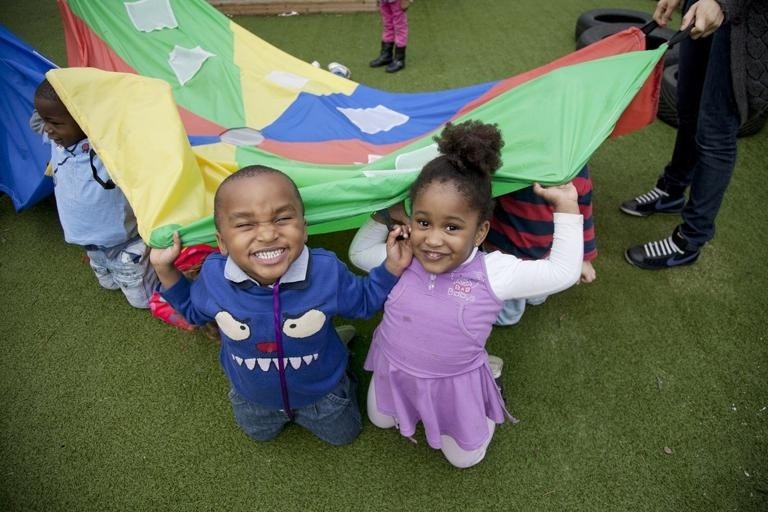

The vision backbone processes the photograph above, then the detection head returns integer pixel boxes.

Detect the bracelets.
[374,209,391,222]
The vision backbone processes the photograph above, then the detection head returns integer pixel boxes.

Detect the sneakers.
[619,187,686,218]
[336,325,357,347]
[624,224,706,271]
[486,355,505,380]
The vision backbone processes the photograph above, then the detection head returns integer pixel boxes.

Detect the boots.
[385,45,406,73]
[369,41,392,67]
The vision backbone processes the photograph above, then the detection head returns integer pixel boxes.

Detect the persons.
[348,120,585,467]
[480,161,598,327]
[28,78,161,310]
[149,164,413,445]
[618,1,767,270]
[368,1,414,73]
[311,59,353,80]
